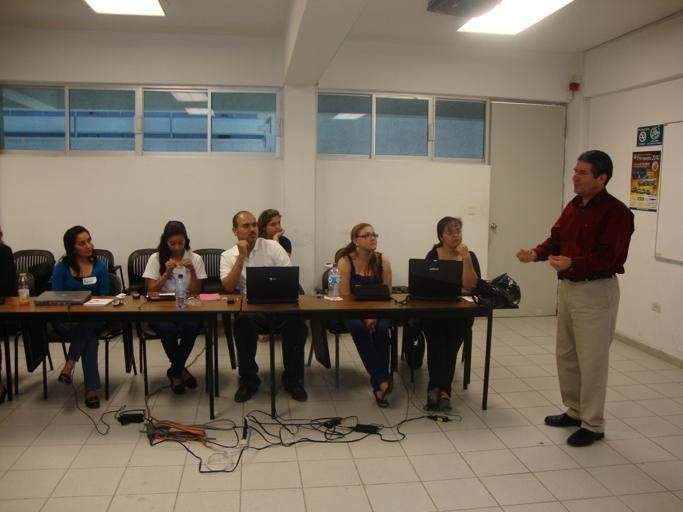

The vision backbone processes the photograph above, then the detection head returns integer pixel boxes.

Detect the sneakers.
[166,368,186,394]
[179,365,198,390]
[234,379,260,403]
[282,378,308,402]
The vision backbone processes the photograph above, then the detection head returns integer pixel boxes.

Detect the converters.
[354,423,379,434]
[432,413,448,423]
[323,417,341,428]
[117,412,144,424]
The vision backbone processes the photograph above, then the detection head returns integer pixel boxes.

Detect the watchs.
[567,261,576,272]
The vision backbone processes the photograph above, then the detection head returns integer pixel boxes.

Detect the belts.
[562,272,613,283]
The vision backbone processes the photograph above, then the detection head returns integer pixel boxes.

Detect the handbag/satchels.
[470,272,522,308]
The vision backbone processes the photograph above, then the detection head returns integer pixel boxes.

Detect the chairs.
[306,267,351,389]
[9,250,57,396]
[128,248,212,398]
[41,249,117,401]
[193,249,231,292]
[400,325,473,389]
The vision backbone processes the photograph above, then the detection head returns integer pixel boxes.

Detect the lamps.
[83,0,167,18]
[456,0,573,37]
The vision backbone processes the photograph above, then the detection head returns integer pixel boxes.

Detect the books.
[83,298,113,307]
[198,293,221,302]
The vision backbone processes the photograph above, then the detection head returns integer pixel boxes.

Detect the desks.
[242,294,520,420]
[0,294,242,420]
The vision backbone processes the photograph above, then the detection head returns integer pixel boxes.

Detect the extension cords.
[238,420,252,446]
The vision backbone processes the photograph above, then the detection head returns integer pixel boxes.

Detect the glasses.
[358,233,379,238]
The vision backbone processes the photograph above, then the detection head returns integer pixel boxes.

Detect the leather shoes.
[85,395,100,408]
[372,389,389,408]
[59,369,72,384]
[545,413,581,427]
[385,375,394,396]
[566,428,605,447]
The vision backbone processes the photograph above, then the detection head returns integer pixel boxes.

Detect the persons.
[219,210,309,404]
[425,216,482,411]
[516,151,635,448]
[142,220,208,396]
[257,208,292,258]
[0,228,18,407]
[336,223,395,408]
[52,226,109,409]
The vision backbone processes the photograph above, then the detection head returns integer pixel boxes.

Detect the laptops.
[245,266,300,304]
[34,290,92,306]
[408,258,462,302]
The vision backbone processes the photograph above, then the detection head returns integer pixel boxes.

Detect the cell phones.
[226,296,234,303]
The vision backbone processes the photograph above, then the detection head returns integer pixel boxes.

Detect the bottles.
[328,264,341,299]
[175,274,186,310]
[18,274,30,306]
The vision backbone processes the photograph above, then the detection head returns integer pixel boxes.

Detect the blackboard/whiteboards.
[654,120,683,264]
